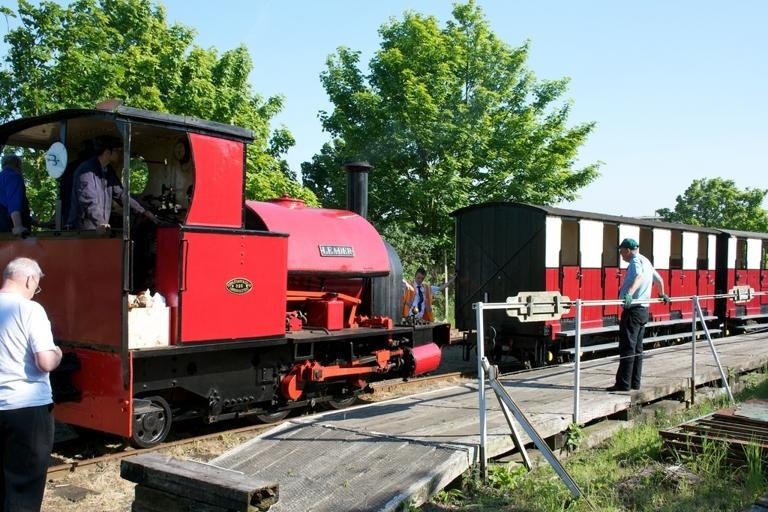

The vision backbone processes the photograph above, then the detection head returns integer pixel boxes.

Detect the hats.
[616,238,639,250]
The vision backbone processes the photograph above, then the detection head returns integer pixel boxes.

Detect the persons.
[0,154,35,238]
[0,257,64,512]
[68,138,158,231]
[409,266,456,319]
[606,238,673,391]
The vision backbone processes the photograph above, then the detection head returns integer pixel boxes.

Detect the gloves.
[659,294,671,305]
[624,294,633,308]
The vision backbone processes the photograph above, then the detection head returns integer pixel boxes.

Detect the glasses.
[32,277,42,295]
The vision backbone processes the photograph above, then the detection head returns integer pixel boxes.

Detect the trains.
[0,97,763,453]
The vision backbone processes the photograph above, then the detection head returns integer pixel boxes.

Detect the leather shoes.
[631,385,640,390]
[606,384,630,391]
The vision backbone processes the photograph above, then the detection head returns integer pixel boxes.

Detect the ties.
[417,285,423,312]
